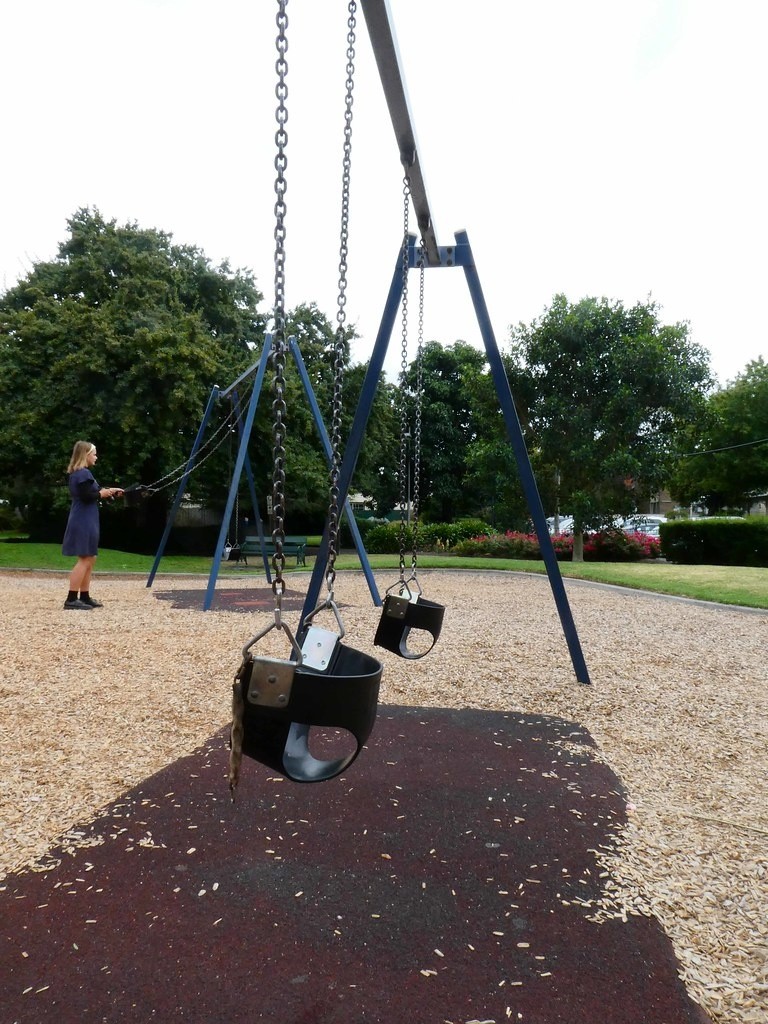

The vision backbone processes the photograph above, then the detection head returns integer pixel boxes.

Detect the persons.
[60,440,124,611]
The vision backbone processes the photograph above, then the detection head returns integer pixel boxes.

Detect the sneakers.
[83,598,103,608]
[63,599,93,610]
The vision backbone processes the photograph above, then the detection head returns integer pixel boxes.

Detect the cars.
[544,511,746,538]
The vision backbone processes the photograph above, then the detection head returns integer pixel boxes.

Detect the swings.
[373,163,445,659]
[117,370,264,503]
[224,0,382,793]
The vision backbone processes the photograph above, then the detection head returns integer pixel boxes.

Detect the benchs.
[240,535,309,568]
